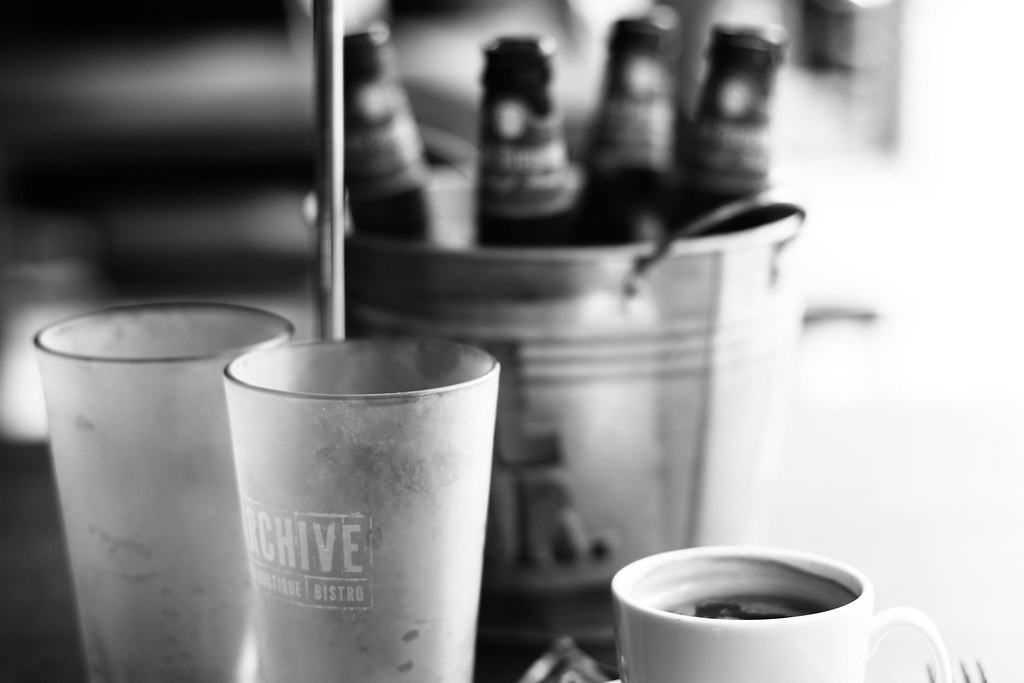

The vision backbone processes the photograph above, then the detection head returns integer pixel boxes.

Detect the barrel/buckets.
[301,174,803,650]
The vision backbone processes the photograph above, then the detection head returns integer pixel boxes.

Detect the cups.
[34,301,293,683]
[222,336,502,683]
[612,544,949,683]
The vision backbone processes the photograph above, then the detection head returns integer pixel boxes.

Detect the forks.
[924,658,991,682]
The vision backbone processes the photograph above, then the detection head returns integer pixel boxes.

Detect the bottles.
[473,35,587,244]
[679,22,788,236]
[342,26,458,239]
[585,16,676,244]
